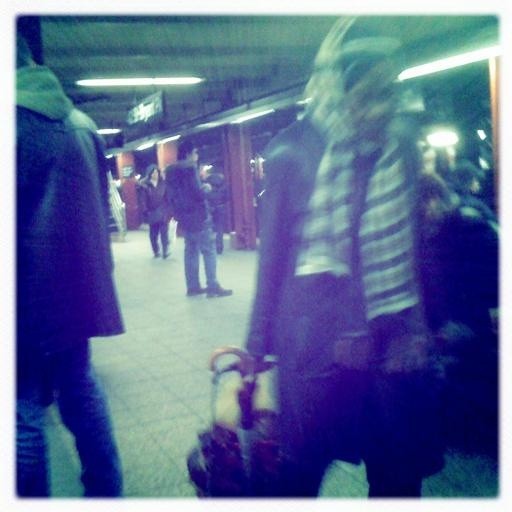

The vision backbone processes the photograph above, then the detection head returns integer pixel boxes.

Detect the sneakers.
[157,251,171,257]
[209,286,231,296]
[186,287,206,295]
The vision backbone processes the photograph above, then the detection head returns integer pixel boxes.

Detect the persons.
[138,165,171,258]
[420,163,500,470]
[245,15,427,499]
[166,141,232,297]
[14,14,127,497]
